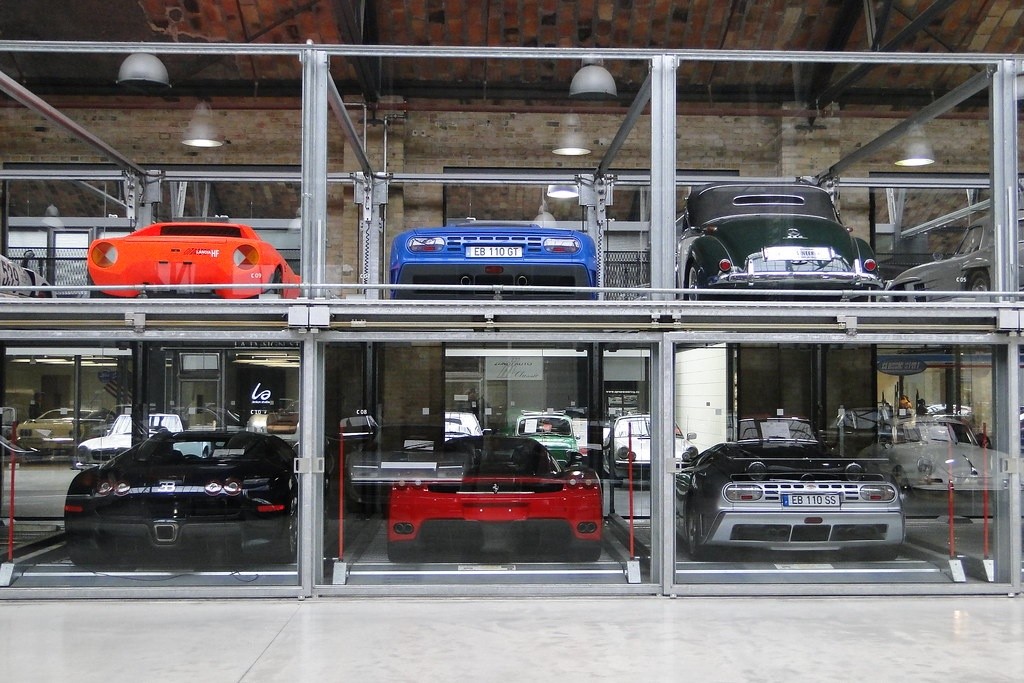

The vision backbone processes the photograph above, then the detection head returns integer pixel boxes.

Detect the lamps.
[115,52,171,95]
[550,114,591,156]
[894,125,935,165]
[181,101,224,148]
[568,59,616,101]
[534,201,554,221]
[545,184,580,198]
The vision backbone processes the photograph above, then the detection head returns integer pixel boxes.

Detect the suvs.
[734,413,832,458]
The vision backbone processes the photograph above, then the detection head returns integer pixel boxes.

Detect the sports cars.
[677,438,907,559]
[385,206,599,301]
[82,216,305,302]
[390,431,605,560]
[61,429,299,568]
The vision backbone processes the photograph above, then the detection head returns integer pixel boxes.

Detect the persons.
[974,433,991,449]
[899,395,912,409]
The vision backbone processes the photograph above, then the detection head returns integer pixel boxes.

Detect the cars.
[608,415,701,488]
[14,403,112,445]
[846,408,1008,517]
[886,194,1024,292]
[514,407,580,473]
[345,421,472,502]
[677,178,885,302]
[262,398,317,433]
[242,412,305,442]
[172,407,245,428]
[73,412,188,468]
[0,247,55,299]
